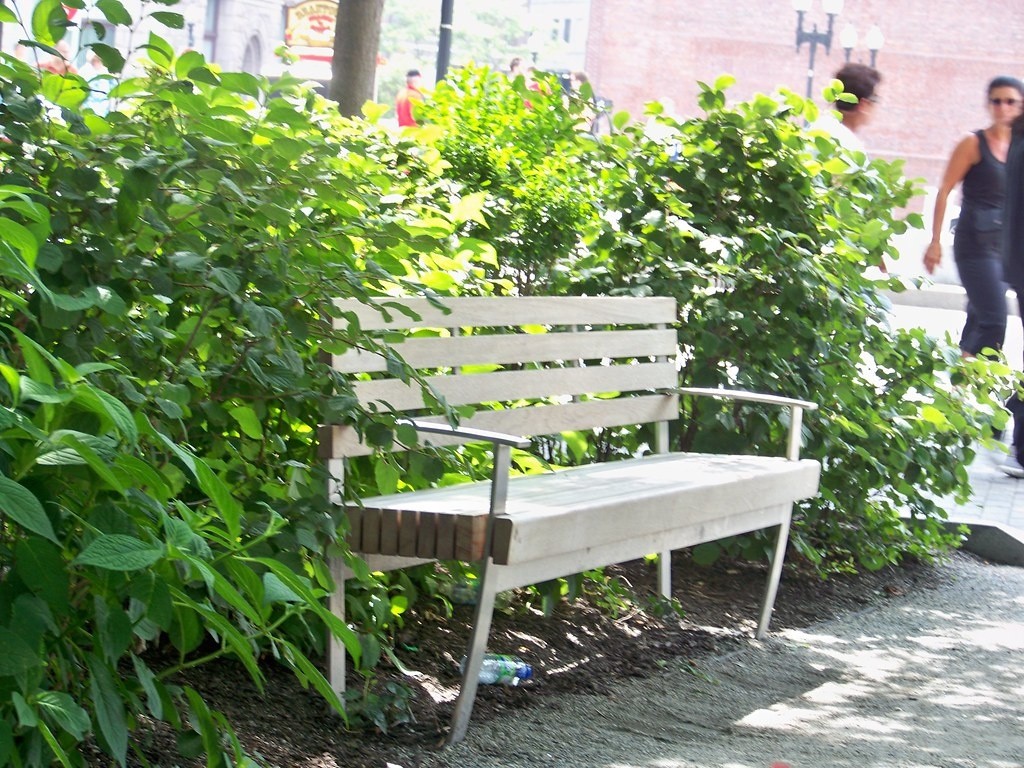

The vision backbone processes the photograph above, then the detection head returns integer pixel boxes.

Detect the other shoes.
[999,445,1024,478]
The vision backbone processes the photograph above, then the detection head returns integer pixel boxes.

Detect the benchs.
[324,294,821,754]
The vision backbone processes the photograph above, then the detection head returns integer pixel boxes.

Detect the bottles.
[459,653,536,686]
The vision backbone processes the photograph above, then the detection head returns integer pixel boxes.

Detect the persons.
[507,57,527,77]
[571,74,588,91]
[396,68,427,126]
[922,75,1024,475]
[822,63,883,164]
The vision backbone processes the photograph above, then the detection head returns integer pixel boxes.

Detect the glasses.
[863,94,881,105]
[988,97,1022,106]
